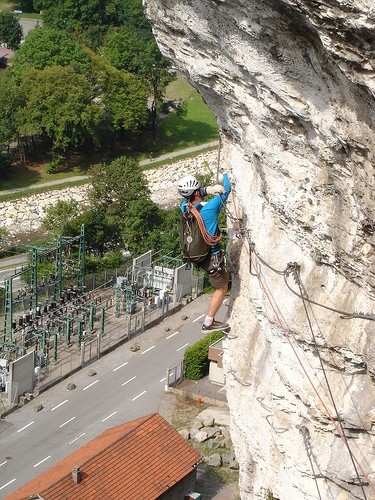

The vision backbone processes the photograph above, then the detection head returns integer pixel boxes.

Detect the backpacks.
[180,201,217,266]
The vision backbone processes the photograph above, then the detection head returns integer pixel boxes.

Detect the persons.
[176,168,231,332]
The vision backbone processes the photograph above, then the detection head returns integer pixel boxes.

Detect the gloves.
[205,183,225,196]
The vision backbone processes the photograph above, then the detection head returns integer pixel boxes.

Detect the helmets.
[177,176,199,198]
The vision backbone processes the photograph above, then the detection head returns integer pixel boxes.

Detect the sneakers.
[200,320,230,333]
